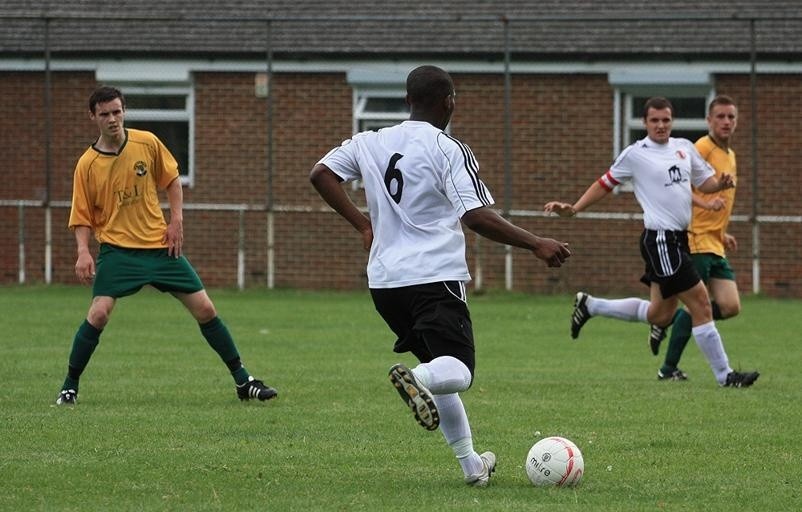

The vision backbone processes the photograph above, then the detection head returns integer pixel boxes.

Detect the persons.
[648,94,740,380]
[310,65,571,489]
[543,93,759,388]
[56,85,279,409]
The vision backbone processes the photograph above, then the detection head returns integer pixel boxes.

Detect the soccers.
[526,437,585,488]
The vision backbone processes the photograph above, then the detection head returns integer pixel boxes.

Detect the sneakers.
[57,389,78,406]
[648,324,667,356]
[656,367,687,381]
[570,292,591,339]
[234,375,278,400]
[720,368,760,388]
[388,363,440,430]
[464,451,496,488]
